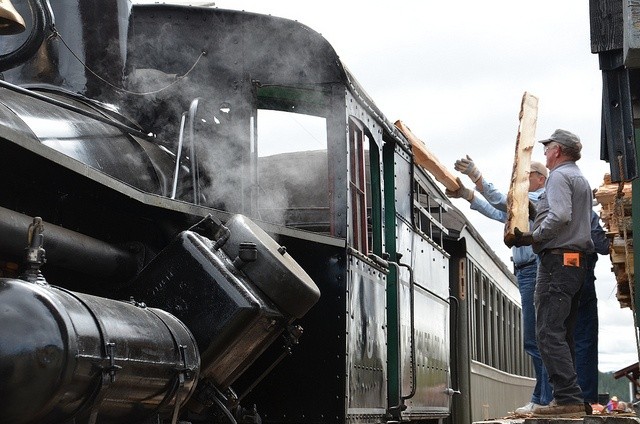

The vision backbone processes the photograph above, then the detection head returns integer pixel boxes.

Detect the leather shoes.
[534,397,588,417]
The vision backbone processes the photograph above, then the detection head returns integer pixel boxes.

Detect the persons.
[445,153,556,416]
[607,393,640,412]
[507,126,611,419]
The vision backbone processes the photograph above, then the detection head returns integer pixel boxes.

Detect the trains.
[0,0,538,424]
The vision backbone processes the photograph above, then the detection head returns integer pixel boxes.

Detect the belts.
[538,248,591,257]
[514,260,536,270]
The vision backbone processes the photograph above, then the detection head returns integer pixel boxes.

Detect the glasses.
[543,146,557,152]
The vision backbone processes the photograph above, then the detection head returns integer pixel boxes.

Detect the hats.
[529,162,547,180]
[538,130,582,151]
[610,396,618,401]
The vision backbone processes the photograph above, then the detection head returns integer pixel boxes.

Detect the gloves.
[454,155,481,183]
[445,177,473,202]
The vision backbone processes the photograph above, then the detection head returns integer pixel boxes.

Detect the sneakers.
[516,404,550,416]
